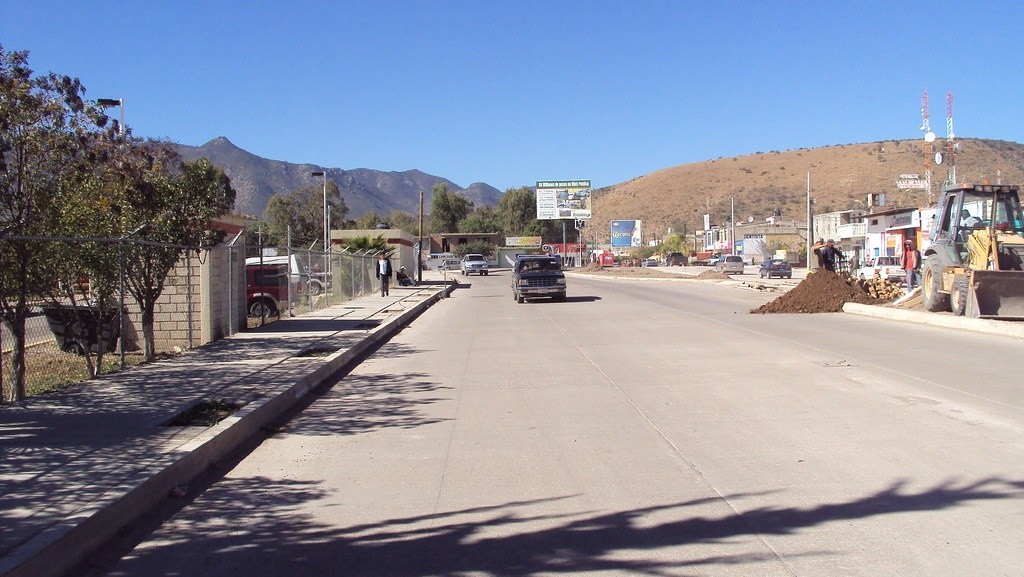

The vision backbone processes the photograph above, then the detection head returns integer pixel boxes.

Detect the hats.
[401,266,406,271]
[827,239,835,243]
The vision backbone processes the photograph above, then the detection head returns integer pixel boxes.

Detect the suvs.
[511,255,566,304]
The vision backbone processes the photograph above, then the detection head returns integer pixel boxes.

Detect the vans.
[598,253,615,267]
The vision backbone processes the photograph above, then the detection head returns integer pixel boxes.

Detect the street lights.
[310,171,328,306]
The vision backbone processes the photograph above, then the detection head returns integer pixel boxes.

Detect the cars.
[859,255,906,287]
[758,260,792,279]
[640,257,659,266]
[243,262,323,321]
[439,253,489,276]
[708,252,745,276]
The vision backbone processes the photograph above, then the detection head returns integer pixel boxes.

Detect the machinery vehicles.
[917,182,1024,321]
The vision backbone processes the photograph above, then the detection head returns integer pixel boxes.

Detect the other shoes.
[386,291,389,296]
[381,293,384,297]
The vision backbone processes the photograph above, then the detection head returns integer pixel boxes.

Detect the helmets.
[815,238,823,243]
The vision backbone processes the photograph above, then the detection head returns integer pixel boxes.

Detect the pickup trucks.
[666,253,688,267]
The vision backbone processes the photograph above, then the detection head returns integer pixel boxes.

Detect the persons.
[958,210,978,238]
[376,252,392,297]
[521,265,529,273]
[810,238,845,273]
[752,257,755,265]
[542,261,555,271]
[397,266,410,286]
[900,240,922,292]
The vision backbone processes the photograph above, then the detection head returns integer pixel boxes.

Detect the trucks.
[688,251,713,266]
